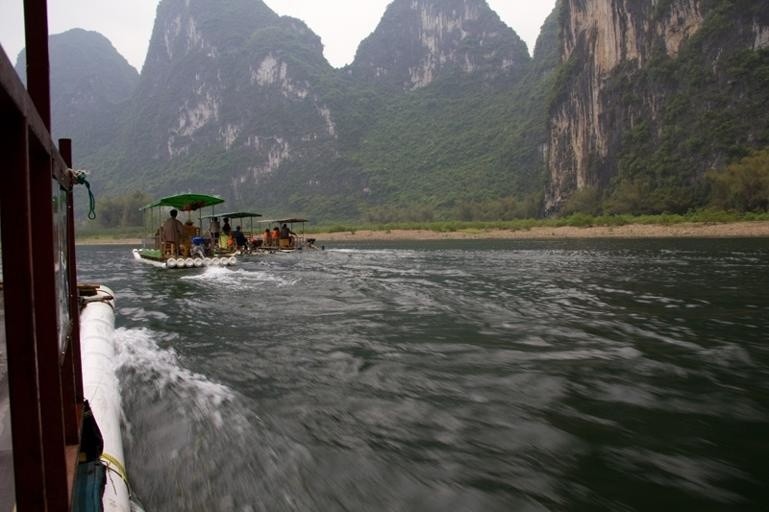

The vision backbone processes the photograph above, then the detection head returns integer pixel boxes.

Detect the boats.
[133,249,303,268]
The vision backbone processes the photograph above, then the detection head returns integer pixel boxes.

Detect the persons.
[209,217,247,250]
[155,209,196,244]
[263,224,296,245]
